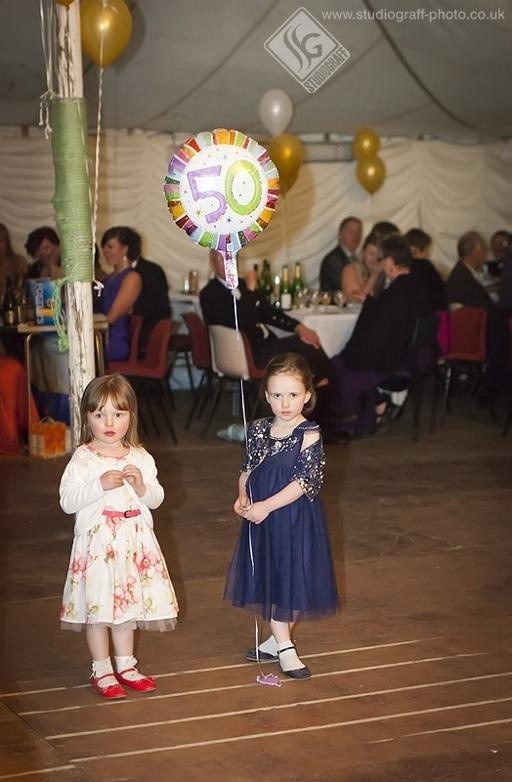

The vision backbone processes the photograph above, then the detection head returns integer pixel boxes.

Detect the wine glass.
[295,287,346,314]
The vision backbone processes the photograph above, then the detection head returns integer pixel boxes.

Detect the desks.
[259,293,361,361]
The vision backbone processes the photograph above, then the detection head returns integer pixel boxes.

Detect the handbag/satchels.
[31,417,73,458]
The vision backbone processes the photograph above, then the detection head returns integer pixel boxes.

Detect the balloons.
[355,152,386,198]
[351,126,381,160]
[79,0,135,72]
[265,131,306,188]
[162,128,282,289]
[258,87,293,141]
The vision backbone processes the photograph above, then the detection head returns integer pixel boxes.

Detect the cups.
[19,295,36,327]
[188,270,198,293]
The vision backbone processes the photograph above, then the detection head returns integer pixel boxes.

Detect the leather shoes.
[370,401,401,436]
[277,638,311,680]
[112,665,158,693]
[89,672,128,699]
[244,647,276,662]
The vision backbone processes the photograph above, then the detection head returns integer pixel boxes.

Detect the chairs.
[381,307,489,443]
[168,305,265,449]
[107,314,179,444]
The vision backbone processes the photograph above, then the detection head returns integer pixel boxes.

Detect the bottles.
[291,262,303,305]
[279,266,293,310]
[3,275,20,325]
[253,259,280,304]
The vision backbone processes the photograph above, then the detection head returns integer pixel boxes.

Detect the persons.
[59,372,181,701]
[222,351,342,680]
[0,217,511,445]
